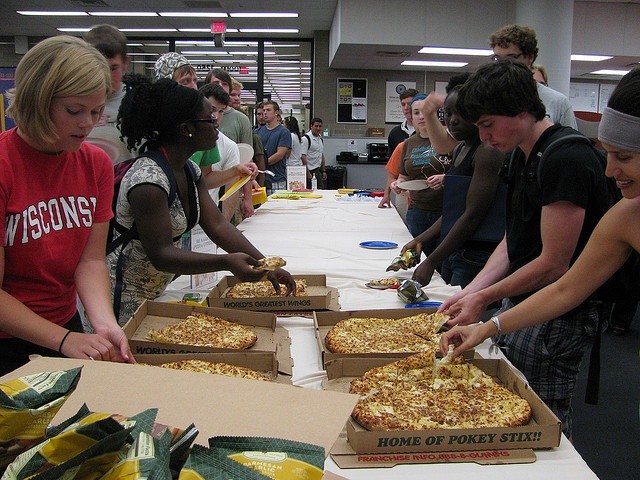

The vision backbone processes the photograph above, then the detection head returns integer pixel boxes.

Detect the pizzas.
[127,355,273,383]
[223,278,311,301]
[251,253,287,273]
[348,342,534,435]
[368,276,403,290]
[323,311,464,355]
[143,309,260,351]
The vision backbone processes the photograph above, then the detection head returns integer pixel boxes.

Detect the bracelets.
[488,315,511,355]
[58,329,73,354]
[322,170,327,173]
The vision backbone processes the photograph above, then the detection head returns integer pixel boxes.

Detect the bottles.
[311,173,317,191]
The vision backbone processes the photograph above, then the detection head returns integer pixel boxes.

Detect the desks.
[87,190,600,480]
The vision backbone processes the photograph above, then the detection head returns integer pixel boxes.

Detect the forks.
[258,169,275,178]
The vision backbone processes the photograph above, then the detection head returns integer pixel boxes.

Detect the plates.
[397,180,430,190]
[358,240,398,249]
[218,174,253,202]
[237,142,254,164]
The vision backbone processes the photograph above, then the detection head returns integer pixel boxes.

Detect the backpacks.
[106,139,198,259]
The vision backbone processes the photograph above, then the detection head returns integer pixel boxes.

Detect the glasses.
[174,114,217,129]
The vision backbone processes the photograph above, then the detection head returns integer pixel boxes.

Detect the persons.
[227,76,245,111]
[532,62,549,87]
[1,32,139,366]
[75,68,298,334]
[300,117,328,190]
[254,100,292,196]
[434,56,604,446]
[397,73,512,290]
[388,87,420,160]
[251,102,266,130]
[389,92,453,260]
[378,141,404,208]
[191,83,242,255]
[418,21,578,155]
[438,65,640,363]
[204,68,255,218]
[413,71,472,163]
[276,113,282,124]
[282,115,304,167]
[152,51,260,250]
[252,133,266,187]
[70,22,149,167]
